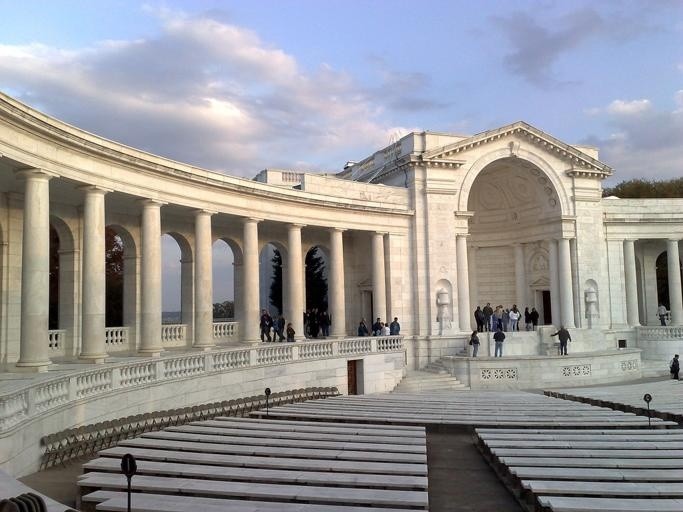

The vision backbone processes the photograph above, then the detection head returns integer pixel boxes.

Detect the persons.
[473,302,522,332]
[530,307,539,331]
[524,306,532,330]
[654,302,667,326]
[672,353,680,380]
[492,328,504,357]
[470,331,480,357]
[257,308,331,342]
[549,325,572,356]
[356,316,400,349]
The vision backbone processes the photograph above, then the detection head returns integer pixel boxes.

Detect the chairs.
[0,491,80,511]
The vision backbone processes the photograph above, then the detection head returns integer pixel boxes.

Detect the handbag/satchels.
[469,340,474,345]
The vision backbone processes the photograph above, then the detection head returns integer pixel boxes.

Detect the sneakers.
[564,353,568,355]
[561,353,564,355]
